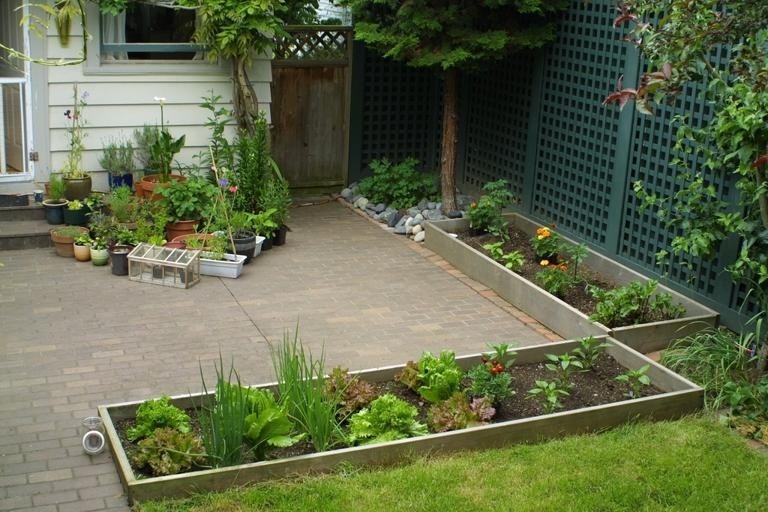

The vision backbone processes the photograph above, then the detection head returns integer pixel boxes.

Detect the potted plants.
[40,123,291,279]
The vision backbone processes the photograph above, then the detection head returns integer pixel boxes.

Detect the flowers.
[153,95,167,131]
[62,82,91,178]
